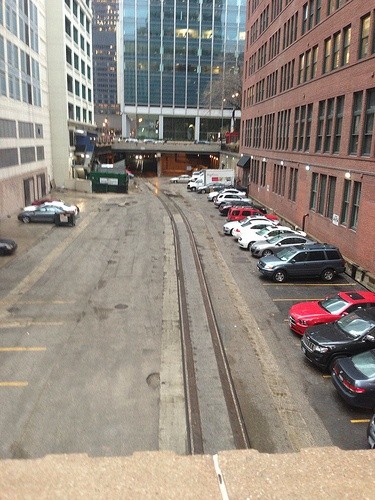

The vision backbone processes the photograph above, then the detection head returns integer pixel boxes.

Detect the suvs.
[227,206,278,222]
[301,307,375,370]
[213,194,253,206]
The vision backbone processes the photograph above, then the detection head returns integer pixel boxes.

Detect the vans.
[256,243,346,283]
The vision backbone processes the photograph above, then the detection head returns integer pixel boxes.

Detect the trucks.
[187,169,234,192]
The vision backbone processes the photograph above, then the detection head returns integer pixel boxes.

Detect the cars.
[32,199,53,206]
[22,201,76,216]
[231,219,278,238]
[218,201,267,214]
[223,216,279,236]
[250,233,318,258]
[288,291,375,335]
[169,174,191,184]
[207,189,247,200]
[17,205,67,224]
[330,348,374,409]
[0,238,17,256]
[197,183,232,194]
[237,226,306,248]
